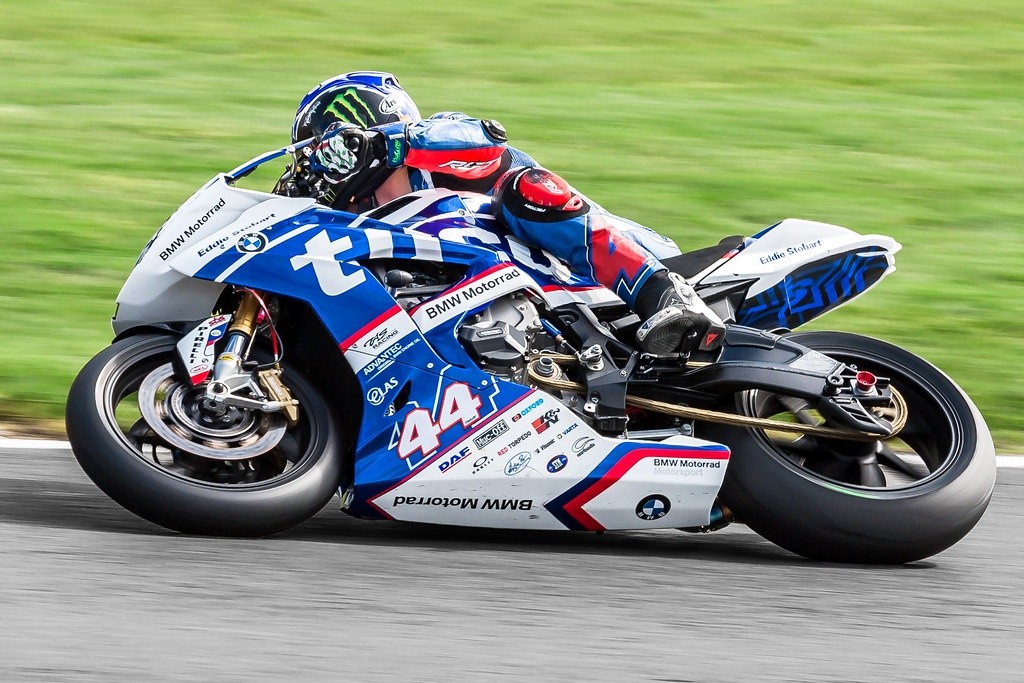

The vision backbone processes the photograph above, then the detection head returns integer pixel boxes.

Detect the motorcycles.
[64,124,999,569]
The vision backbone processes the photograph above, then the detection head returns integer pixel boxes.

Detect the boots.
[634,271,726,355]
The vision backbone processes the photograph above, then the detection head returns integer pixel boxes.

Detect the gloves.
[308,121,409,185]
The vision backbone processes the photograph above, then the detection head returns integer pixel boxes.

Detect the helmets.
[291,71,422,204]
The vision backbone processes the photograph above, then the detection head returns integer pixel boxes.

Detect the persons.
[289,69,726,359]
